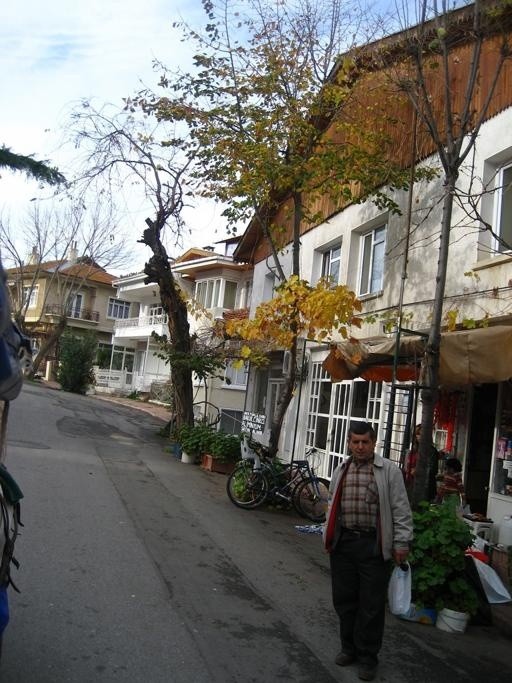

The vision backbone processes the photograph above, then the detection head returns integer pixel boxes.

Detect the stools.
[462,515,493,541]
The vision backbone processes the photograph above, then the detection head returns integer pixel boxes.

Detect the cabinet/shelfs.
[484,380,512,544]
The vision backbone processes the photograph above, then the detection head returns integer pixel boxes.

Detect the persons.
[322,421,413,681]
[403,423,439,509]
[438,459,468,507]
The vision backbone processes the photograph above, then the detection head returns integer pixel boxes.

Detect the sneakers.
[358,662,377,681]
[335,652,357,666]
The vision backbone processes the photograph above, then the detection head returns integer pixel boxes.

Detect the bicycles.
[226,447,330,523]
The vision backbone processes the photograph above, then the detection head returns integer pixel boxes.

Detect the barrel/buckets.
[435,609,471,635]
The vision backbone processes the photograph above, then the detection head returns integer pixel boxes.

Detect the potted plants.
[201,428,237,472]
[179,422,202,464]
